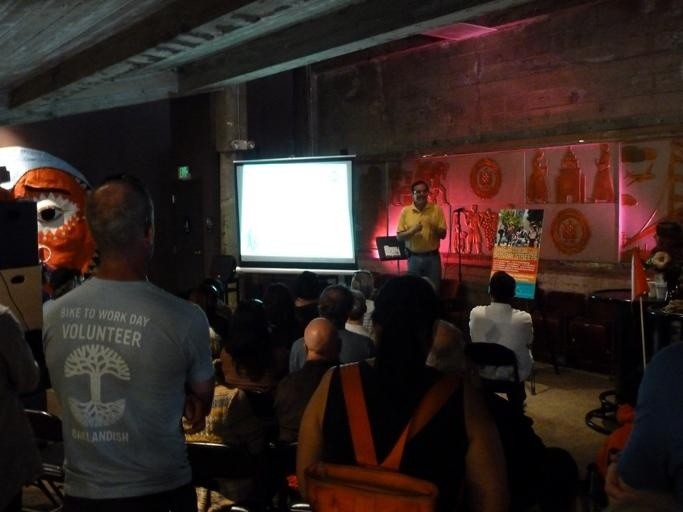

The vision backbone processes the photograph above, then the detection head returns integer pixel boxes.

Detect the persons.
[0,303,43,512]
[498,222,538,248]
[606,341,683,512]
[586,144,616,203]
[191,272,578,511]
[463,205,484,256]
[42,179,216,512]
[527,150,550,204]
[395,181,448,295]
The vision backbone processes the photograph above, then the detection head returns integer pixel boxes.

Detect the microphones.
[453,207,463,212]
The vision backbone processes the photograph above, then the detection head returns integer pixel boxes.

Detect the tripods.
[526,281,561,380]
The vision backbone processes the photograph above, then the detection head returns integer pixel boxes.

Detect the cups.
[648,282,656,297]
[656,283,667,299]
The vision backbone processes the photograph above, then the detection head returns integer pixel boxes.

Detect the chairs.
[15,334,537,512]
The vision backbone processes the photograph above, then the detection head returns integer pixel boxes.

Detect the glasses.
[413,189,426,194]
[109,171,153,223]
